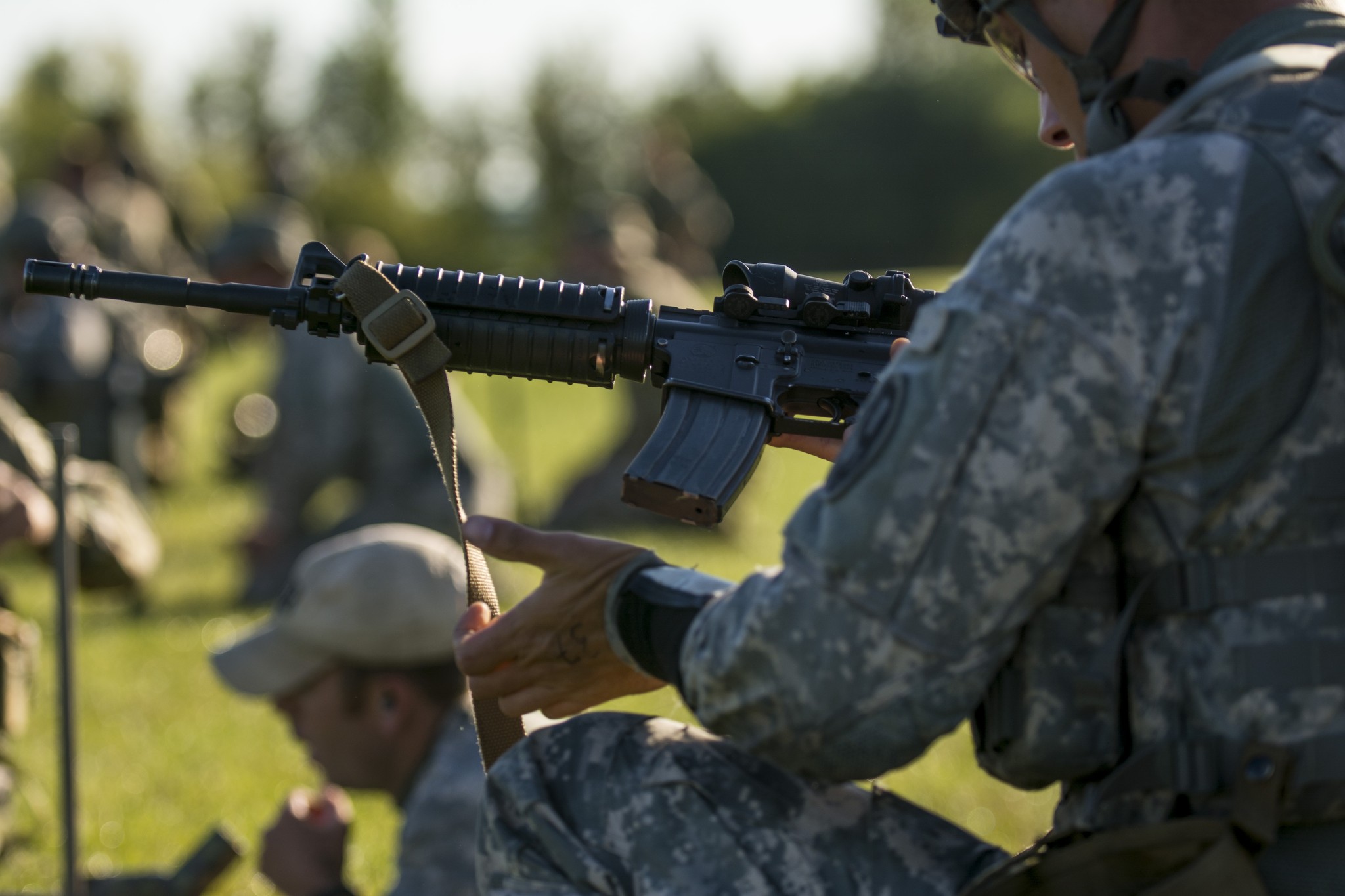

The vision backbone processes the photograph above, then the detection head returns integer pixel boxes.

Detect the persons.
[448,0,1345,896]
[209,522,561,896]
[0,84,745,654]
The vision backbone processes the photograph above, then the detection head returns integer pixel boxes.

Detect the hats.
[203,522,499,703]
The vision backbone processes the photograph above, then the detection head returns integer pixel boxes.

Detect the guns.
[15,238,952,530]
